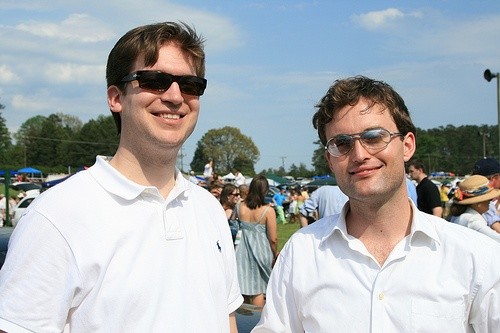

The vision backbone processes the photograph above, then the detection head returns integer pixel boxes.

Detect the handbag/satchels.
[228,207,239,242]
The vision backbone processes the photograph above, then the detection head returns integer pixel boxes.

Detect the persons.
[0,21,244,333]
[249,75,500,333]
[17,173,27,182]
[220,176,278,307]
[0,193,27,228]
[209,173,350,228]
[405,159,500,243]
[188,159,215,187]
[233,170,245,188]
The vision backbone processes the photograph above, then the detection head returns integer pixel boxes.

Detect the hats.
[471,156,500,175]
[17,193,24,198]
[448,175,500,204]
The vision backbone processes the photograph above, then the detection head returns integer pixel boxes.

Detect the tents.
[0,168,42,178]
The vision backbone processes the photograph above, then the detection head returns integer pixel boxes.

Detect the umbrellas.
[218,168,336,189]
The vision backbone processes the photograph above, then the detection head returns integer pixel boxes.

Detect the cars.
[8,181,41,227]
[264,186,289,204]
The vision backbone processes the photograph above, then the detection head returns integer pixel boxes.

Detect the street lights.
[483,69,500,165]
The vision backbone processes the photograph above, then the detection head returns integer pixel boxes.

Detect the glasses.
[122,69,208,97]
[325,127,403,157]
[232,192,240,196]
